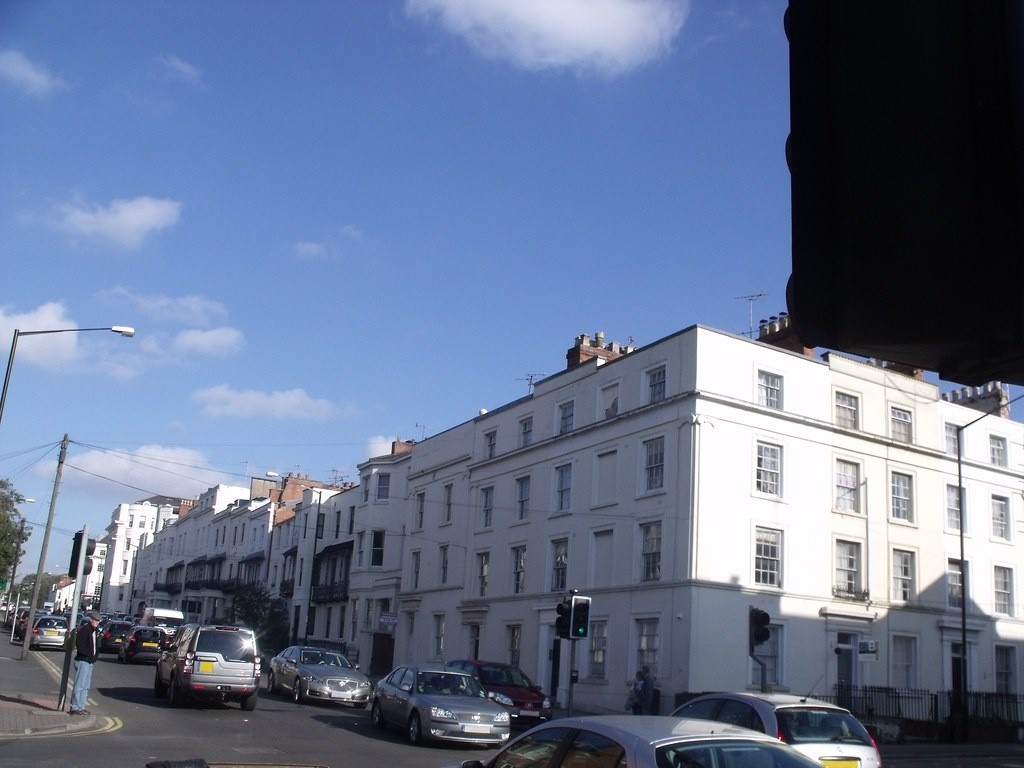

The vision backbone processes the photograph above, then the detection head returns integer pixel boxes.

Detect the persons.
[630,671,644,715]
[637,666,655,715]
[69,612,102,715]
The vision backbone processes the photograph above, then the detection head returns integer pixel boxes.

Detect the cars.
[456,712,823,768]
[267,646,371,709]
[370,663,510,748]
[669,689,882,768]
[444,657,553,725]
[1,597,186,664]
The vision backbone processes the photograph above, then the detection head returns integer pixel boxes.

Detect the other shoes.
[70,710,90,715]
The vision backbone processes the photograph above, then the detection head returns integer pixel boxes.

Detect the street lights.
[0,324,136,432]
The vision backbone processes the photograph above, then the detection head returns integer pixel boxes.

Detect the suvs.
[155,622,261,712]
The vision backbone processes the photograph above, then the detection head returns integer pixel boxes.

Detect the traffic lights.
[752,608,772,647]
[555,599,570,640]
[570,598,591,639]
[82,537,96,575]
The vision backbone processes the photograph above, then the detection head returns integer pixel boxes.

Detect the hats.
[89,611,102,620]
